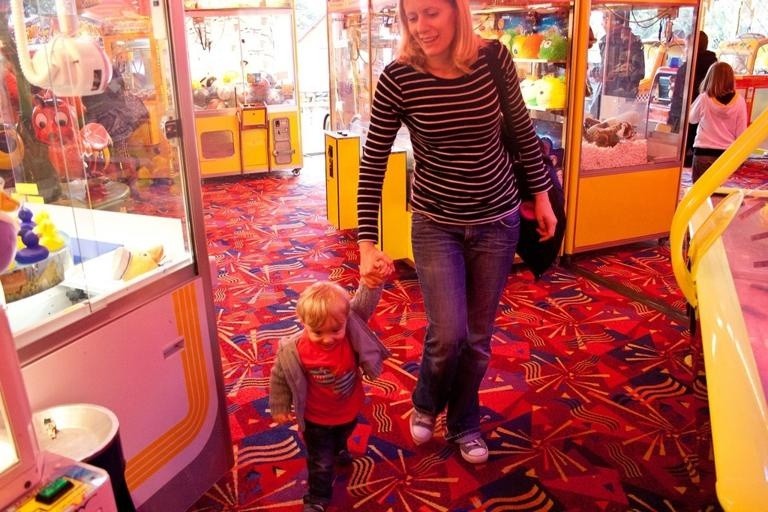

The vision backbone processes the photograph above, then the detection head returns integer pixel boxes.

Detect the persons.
[667,30,747,184]
[589,7,645,122]
[357,0,558,463]
[269,261,392,512]
[82,50,153,202]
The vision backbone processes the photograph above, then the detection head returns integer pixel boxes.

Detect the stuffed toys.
[115,245,163,280]
[192,73,294,109]
[583,118,638,148]
[31,94,112,209]
[480,23,566,109]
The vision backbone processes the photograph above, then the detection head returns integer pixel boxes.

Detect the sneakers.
[336,450,353,465]
[458,438,490,465]
[304,502,327,512]
[410,408,437,443]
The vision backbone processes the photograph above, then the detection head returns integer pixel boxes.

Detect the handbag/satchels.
[513,156,568,283]
